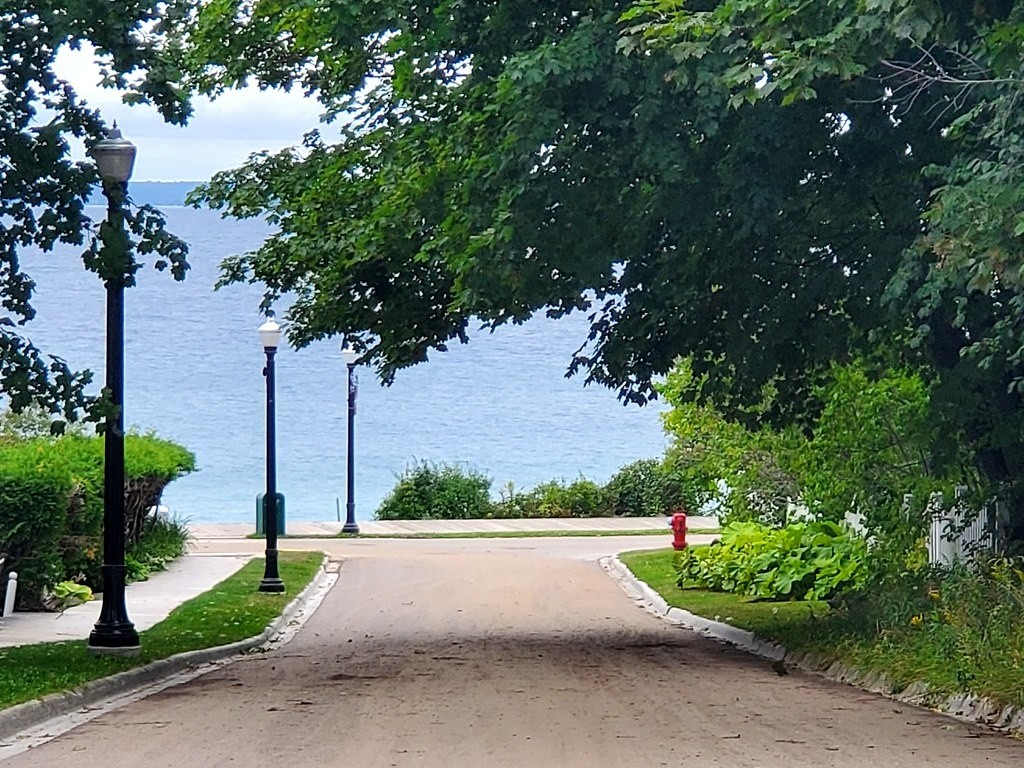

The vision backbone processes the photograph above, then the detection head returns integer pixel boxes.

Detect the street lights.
[258,316,286,595]
[340,342,362,534]
[87,118,143,656]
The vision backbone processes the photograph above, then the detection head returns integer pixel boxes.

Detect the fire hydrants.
[663,515,693,550]
